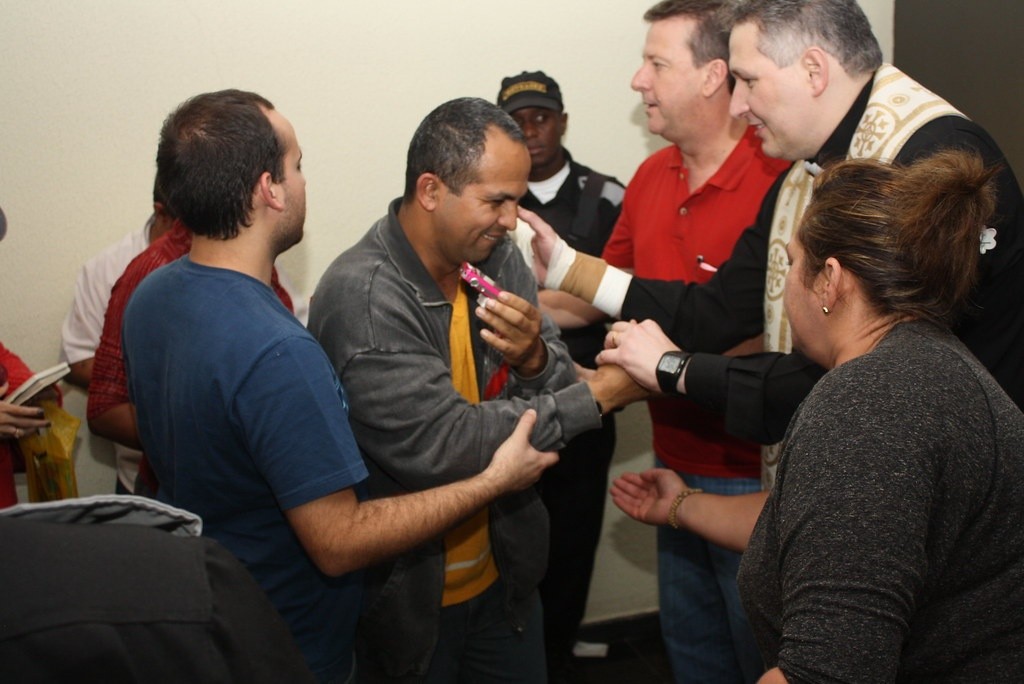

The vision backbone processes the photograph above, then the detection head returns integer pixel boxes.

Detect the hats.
[497,71,564,116]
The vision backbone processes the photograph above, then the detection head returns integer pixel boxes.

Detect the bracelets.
[669,488,704,528]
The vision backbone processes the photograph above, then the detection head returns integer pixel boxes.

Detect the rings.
[612,332,618,345]
[15,429,19,438]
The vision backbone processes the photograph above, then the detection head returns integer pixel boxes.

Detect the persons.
[0,496,303,684]
[507,1,1024,493]
[123,90,560,684]
[608,146,1024,684]
[61,172,307,496]
[307,97,650,684]
[87,222,294,451]
[537,0,795,684]
[497,70,626,684]
[0,209,64,512]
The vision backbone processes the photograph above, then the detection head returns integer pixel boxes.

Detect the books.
[6,362,72,406]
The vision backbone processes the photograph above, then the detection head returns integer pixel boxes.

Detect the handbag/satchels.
[18,401,80,501]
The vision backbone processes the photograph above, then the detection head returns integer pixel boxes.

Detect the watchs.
[656,351,692,394]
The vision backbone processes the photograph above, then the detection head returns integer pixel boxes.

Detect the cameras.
[459,262,502,299]
